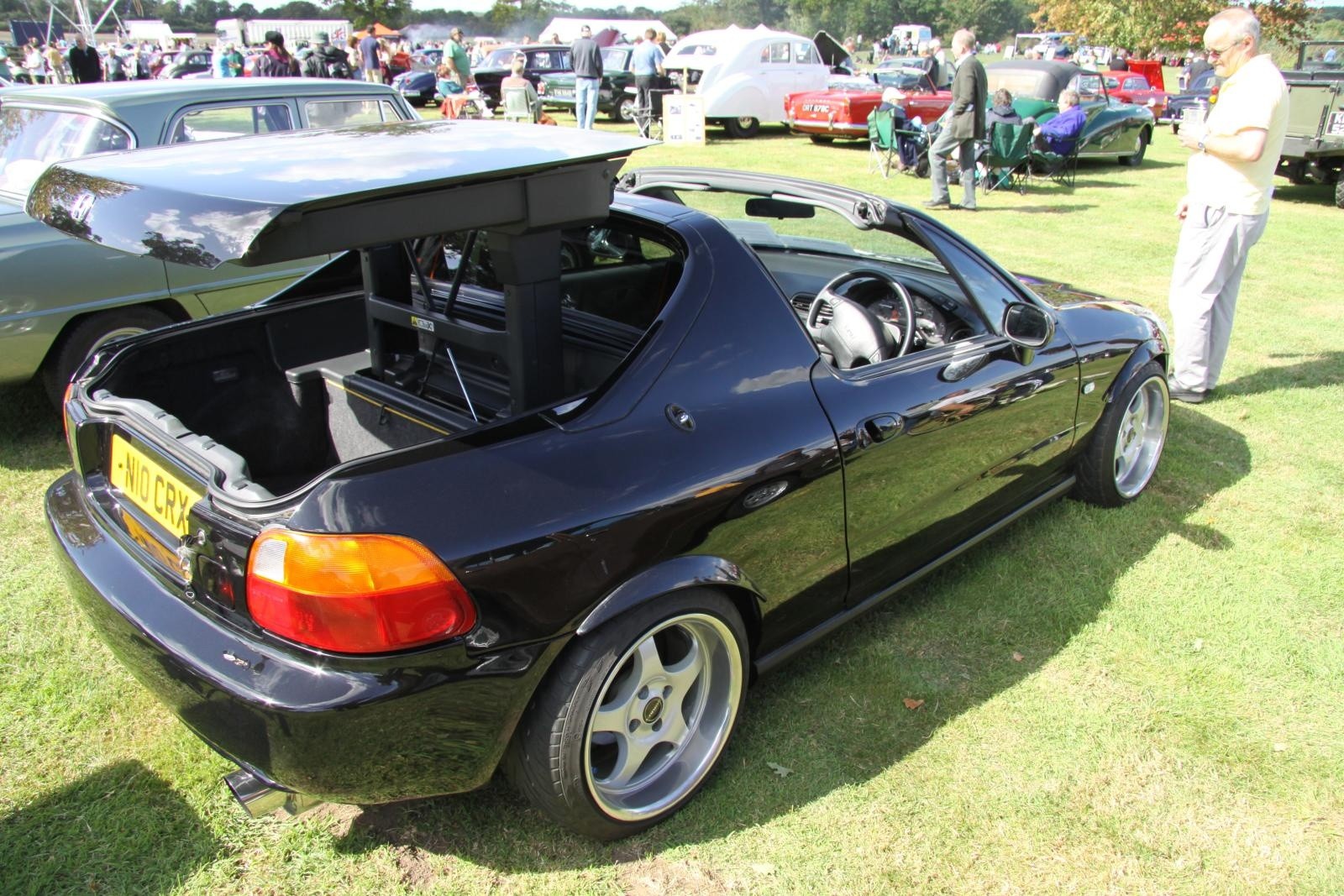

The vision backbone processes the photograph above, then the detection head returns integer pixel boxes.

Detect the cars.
[433,44,576,110]
[967,60,1157,170]
[390,48,451,105]
[535,44,674,124]
[874,59,958,96]
[1,76,427,411]
[101,38,272,80]
[1167,68,1224,135]
[22,118,1185,845]
[1103,60,1176,122]
[783,67,955,145]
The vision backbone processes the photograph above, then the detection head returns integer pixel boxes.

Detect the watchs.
[1198,140,1205,151]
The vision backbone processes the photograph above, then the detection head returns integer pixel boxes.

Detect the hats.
[881,86,907,100]
[260,31,284,46]
[310,31,330,46]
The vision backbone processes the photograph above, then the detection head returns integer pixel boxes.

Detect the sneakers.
[1166,372,1213,403]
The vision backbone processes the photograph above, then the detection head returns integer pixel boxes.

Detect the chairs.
[434,81,474,120]
[499,87,538,122]
[623,88,675,142]
[867,104,1102,195]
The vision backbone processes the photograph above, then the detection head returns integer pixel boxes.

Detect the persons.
[839,31,917,77]
[1315,46,1336,63]
[253,25,360,134]
[935,367,1054,420]
[1111,48,1128,71]
[762,43,790,62]
[975,88,1022,190]
[1024,89,1086,155]
[832,427,868,458]
[976,40,1000,54]
[356,25,688,139]
[0,24,244,85]
[1167,7,1289,404]
[1064,50,1098,72]
[879,89,943,176]
[1023,48,1043,60]
[1117,45,1213,90]
[918,29,989,210]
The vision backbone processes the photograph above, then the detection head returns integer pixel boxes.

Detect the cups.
[1182,109,1207,124]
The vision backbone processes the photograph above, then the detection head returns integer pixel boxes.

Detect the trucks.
[215,16,354,51]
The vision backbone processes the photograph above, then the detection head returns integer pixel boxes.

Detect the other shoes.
[921,199,951,209]
[949,202,977,211]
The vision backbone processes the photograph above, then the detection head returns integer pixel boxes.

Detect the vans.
[654,25,852,139]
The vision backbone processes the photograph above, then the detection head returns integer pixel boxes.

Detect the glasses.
[454,31,464,35]
[1204,40,1244,59]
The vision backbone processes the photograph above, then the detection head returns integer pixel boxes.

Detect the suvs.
[1282,41,1344,207]
